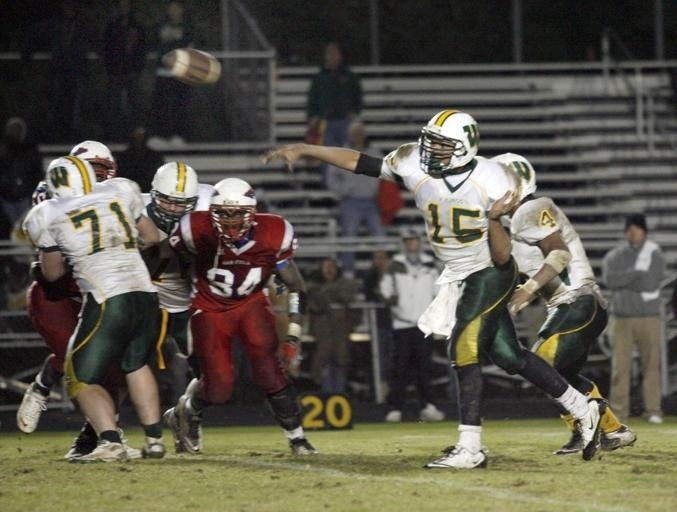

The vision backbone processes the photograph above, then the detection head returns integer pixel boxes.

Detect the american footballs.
[161,48,223,86]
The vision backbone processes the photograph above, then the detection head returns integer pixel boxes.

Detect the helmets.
[150,162,199,224]
[209,178,258,244]
[45,140,118,198]
[490,153,537,207]
[419,110,481,175]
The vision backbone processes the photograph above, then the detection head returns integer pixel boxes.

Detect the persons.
[263,43,667,470]
[0,118,321,462]
[1,1,229,143]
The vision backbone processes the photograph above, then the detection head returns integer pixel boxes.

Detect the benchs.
[37,61,676,284]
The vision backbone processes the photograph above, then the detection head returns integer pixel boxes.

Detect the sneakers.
[66,395,201,464]
[16,382,51,434]
[423,444,488,469]
[290,437,318,456]
[554,398,636,461]
